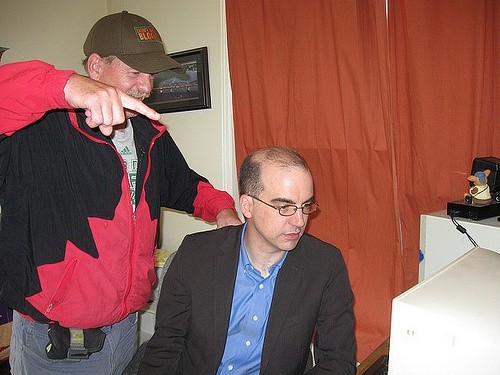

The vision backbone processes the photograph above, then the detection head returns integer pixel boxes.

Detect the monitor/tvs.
[388,247,500,375]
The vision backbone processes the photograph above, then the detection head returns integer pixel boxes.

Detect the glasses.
[248,195,319,216]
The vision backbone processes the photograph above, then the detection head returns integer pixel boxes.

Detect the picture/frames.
[142,46,212,115]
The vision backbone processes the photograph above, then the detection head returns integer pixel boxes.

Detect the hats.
[83,11,182,73]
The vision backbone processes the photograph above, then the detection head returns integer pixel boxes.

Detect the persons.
[137,146,358,375]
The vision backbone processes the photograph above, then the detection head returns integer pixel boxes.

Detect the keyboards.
[362,355,389,375]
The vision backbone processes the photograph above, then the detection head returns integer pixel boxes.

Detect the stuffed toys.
[467,168,492,205]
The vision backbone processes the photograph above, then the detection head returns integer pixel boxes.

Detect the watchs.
[0,10,240,375]
